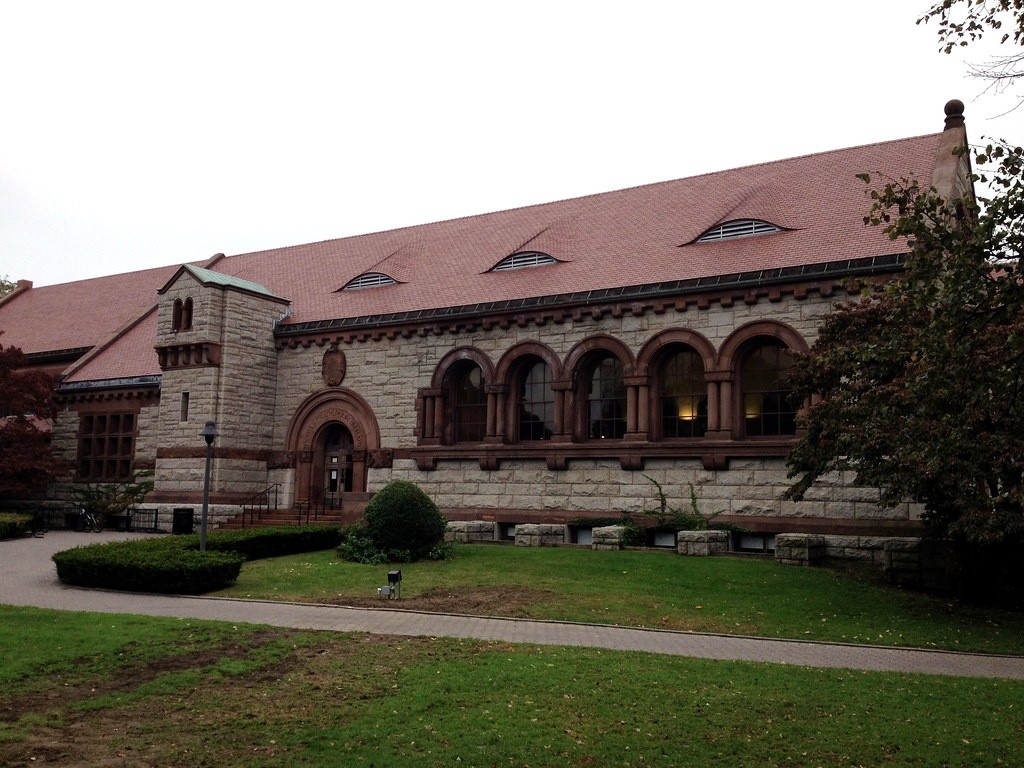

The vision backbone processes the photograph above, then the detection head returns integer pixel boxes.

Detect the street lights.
[198,421,222,551]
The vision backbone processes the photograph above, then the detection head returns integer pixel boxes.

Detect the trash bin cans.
[171,507,195,535]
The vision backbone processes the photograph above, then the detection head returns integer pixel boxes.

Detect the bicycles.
[77,498,104,533]
